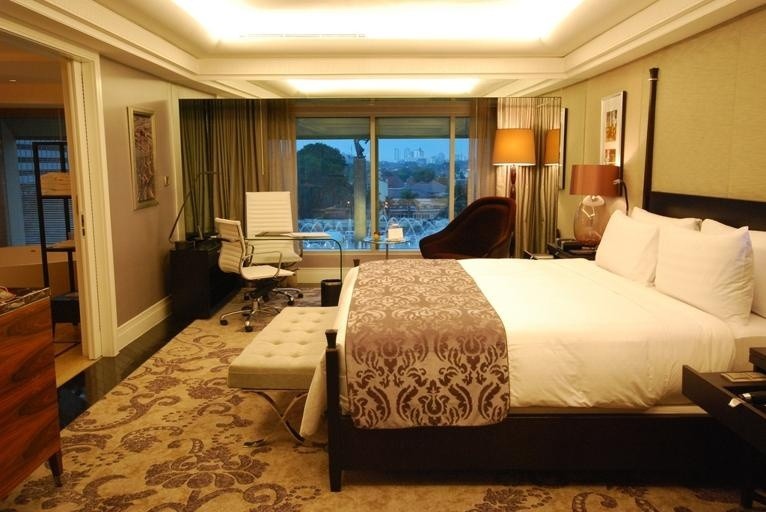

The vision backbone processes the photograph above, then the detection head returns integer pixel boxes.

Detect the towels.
[37,167,70,197]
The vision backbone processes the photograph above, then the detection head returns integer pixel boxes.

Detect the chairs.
[417,194,518,259]
[242,189,307,307]
[211,215,296,332]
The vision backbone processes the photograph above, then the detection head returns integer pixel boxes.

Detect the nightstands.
[680,342,765,511]
[522,239,598,260]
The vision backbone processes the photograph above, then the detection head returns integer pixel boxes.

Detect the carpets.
[0,281,764,511]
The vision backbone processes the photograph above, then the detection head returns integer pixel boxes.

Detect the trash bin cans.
[321,279,342,306]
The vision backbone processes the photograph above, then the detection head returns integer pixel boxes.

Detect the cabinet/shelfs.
[0,287,69,505]
[168,235,243,320]
[29,138,84,344]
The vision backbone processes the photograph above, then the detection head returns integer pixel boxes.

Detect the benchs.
[225,300,341,450]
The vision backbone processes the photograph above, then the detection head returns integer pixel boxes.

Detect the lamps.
[490,126,536,258]
[568,162,622,251]
[542,127,560,168]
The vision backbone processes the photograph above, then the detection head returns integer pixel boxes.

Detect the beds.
[322,66,765,493]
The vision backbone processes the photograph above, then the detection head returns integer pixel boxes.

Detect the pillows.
[591,209,666,285]
[701,215,766,322]
[630,204,703,234]
[654,218,756,327]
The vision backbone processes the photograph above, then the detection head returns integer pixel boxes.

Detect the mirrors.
[532,88,562,254]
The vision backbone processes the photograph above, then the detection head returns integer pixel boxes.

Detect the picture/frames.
[123,104,161,212]
[598,90,626,168]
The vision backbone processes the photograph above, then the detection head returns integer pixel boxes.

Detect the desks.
[359,231,411,260]
[240,229,344,304]
